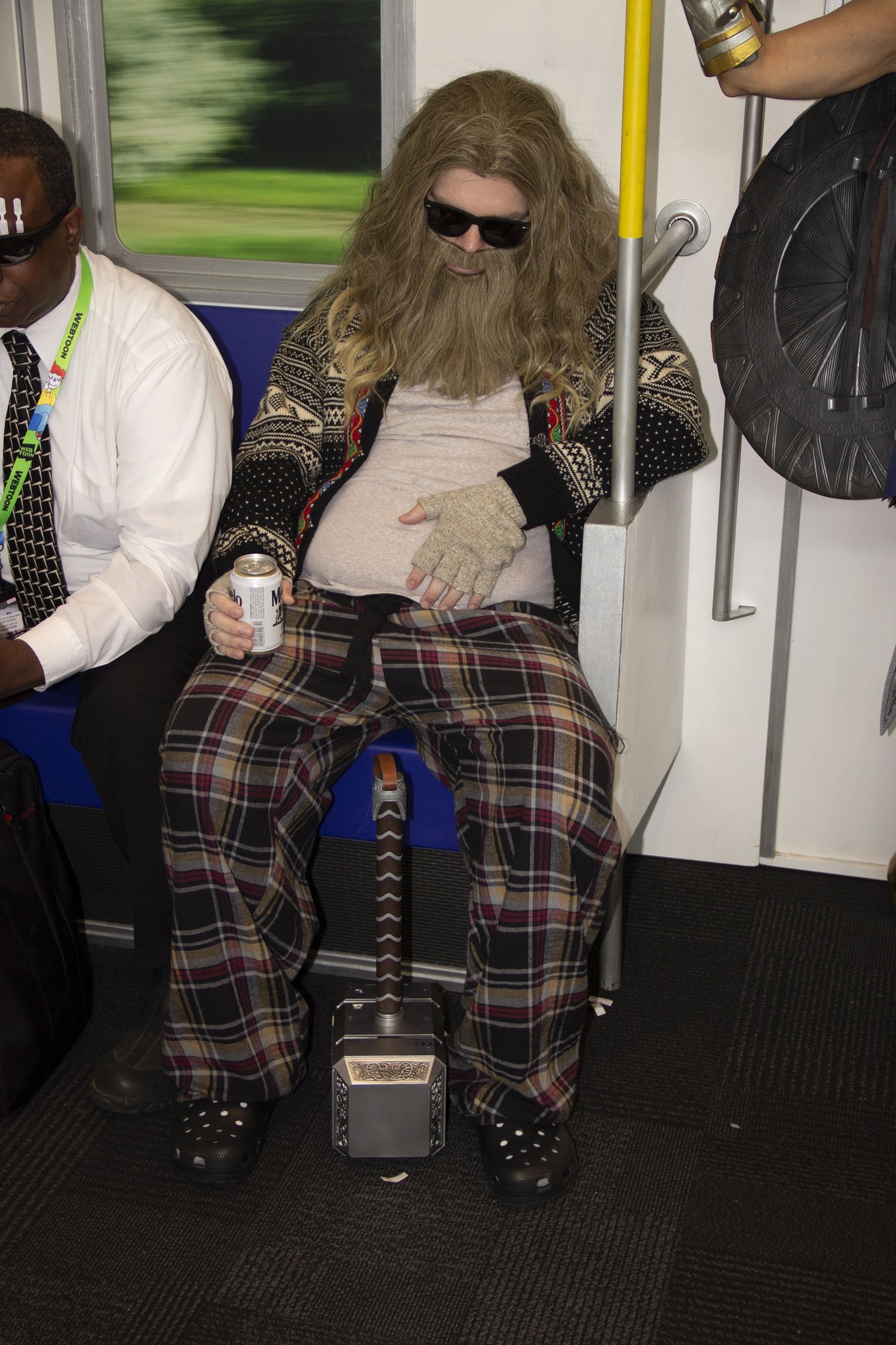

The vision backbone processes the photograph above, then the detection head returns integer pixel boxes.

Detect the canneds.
[229,554,284,658]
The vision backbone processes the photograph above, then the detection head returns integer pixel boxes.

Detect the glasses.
[0,201,76,266]
[424,195,532,249]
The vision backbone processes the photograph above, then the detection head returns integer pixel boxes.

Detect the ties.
[2,329,68,629]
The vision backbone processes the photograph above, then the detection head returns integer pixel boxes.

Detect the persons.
[679,0,896,100]
[0,108,234,1114]
[159,70,717,1205]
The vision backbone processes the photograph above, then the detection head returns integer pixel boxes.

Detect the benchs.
[0,295,685,1020]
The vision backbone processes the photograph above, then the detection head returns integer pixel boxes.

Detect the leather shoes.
[169,1101,270,1181]
[482,1121,576,1202]
[89,966,179,1115]
